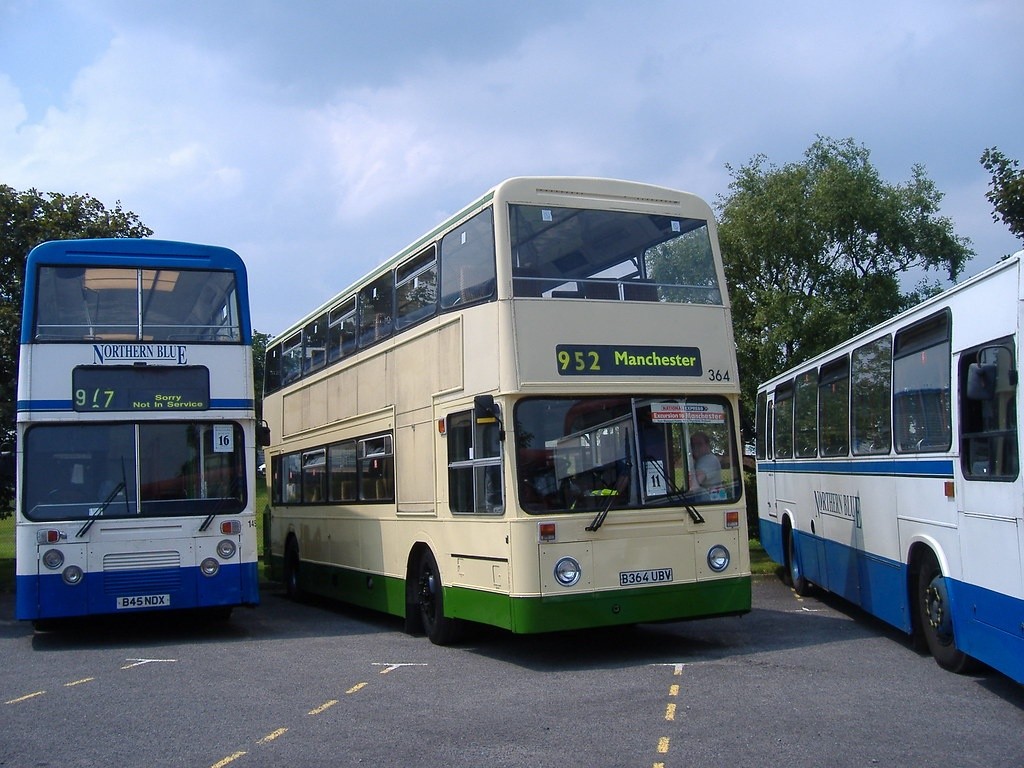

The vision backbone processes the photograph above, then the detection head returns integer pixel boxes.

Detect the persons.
[688,432,722,488]
[857,419,889,454]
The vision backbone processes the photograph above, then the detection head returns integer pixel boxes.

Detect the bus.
[260,175,755,646]
[750,248,1024,688]
[0,234,272,633]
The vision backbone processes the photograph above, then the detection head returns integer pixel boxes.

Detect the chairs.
[309,478,390,501]
[779,442,892,457]
[285,279,656,386]
